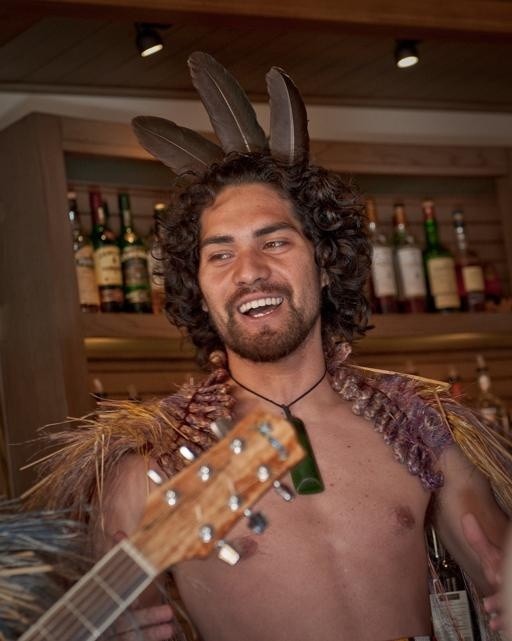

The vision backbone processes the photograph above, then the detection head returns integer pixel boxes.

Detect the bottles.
[66,187,170,315]
[364,196,486,316]
[448,355,512,433]
[427,521,497,641]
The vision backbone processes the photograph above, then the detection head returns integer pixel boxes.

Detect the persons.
[89,155,512,641]
[0,509,175,639]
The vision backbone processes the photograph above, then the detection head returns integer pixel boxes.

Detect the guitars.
[13,408,308,641]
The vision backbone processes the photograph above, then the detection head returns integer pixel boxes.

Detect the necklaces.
[225,370,331,494]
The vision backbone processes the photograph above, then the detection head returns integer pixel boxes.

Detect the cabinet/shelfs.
[1,110,511,510]
